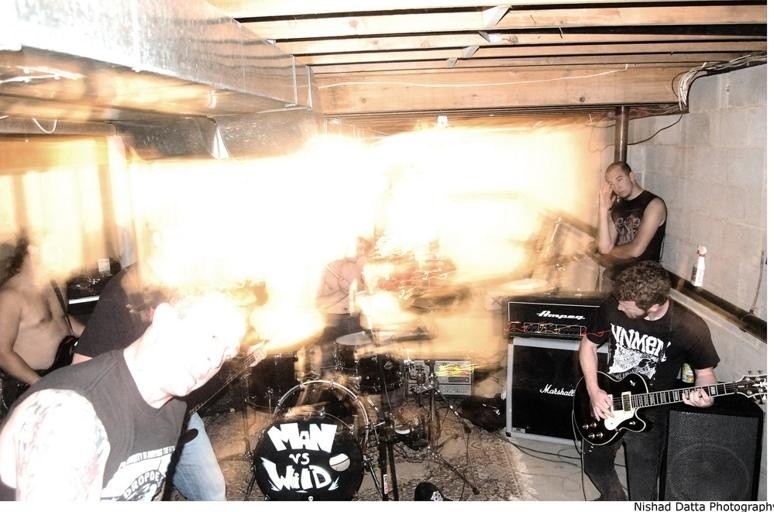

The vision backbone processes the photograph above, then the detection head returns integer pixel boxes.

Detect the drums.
[355,343,406,395]
[244,379,370,501]
[331,329,394,376]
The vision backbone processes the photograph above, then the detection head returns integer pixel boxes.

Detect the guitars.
[1,334,79,411]
[574,370,768,448]
[182,341,274,414]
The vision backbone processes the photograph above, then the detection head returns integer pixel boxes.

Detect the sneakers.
[592,494,624,502]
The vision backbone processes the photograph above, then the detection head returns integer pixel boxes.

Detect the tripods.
[422,359,471,449]
[370,348,481,501]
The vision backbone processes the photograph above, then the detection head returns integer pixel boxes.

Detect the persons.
[0,289,246,502]
[316,256,363,334]
[578,260,720,499]
[0,244,87,388]
[72,265,227,500]
[587,163,667,295]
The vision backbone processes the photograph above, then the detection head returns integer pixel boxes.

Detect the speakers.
[659,379,765,500]
